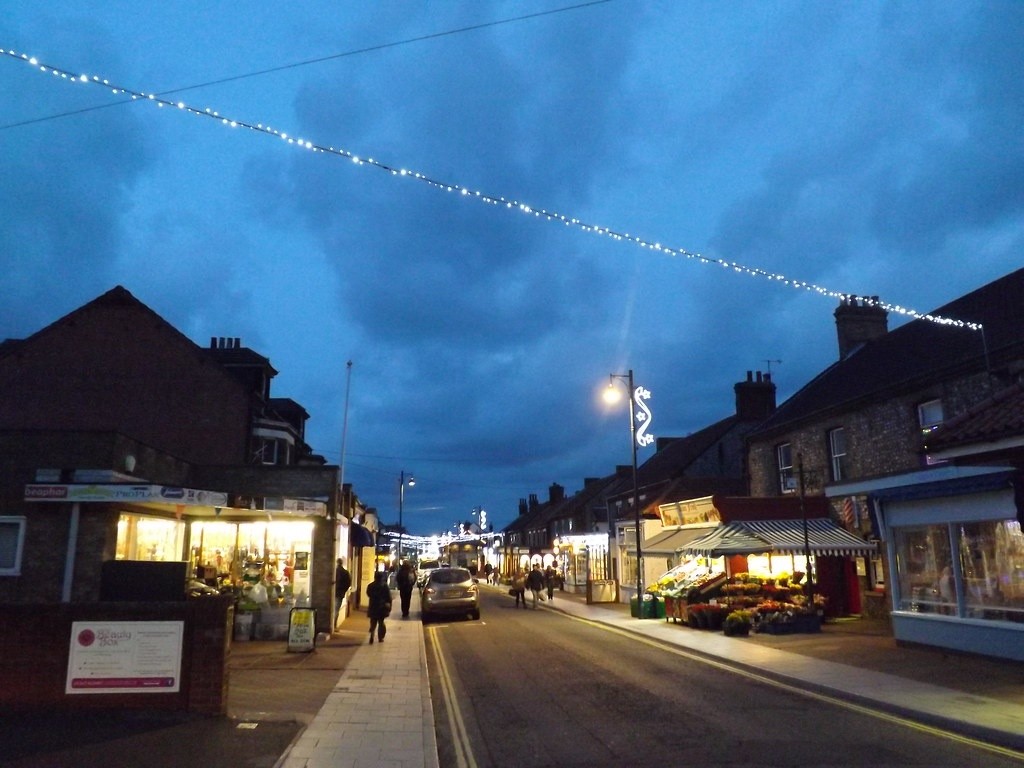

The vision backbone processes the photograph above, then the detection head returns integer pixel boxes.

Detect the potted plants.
[722,610,752,637]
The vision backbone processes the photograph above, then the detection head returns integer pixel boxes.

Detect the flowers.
[685,603,720,615]
[715,570,828,625]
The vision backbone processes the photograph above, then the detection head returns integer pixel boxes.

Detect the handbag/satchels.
[539,593,545,601]
[408,568,414,584]
[509,589,517,596]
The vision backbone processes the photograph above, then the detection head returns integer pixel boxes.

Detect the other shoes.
[379,639,384,642]
[370,637,374,644]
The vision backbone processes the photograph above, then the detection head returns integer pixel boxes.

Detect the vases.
[694,613,719,630]
[795,615,820,632]
[770,622,794,635]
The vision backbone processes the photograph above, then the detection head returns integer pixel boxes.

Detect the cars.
[419,566,480,624]
[417,559,443,588]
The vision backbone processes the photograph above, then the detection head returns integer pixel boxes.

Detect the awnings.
[626,519,876,558]
[352,524,375,548]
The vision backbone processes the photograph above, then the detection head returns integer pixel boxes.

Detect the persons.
[940,567,956,604]
[367,572,393,645]
[335,558,353,630]
[492,565,500,586]
[512,567,528,609]
[397,560,417,619]
[527,565,545,610]
[484,561,492,584]
[545,565,555,599]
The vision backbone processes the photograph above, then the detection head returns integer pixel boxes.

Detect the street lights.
[601,367,644,620]
[472,504,480,539]
[397,469,417,569]
